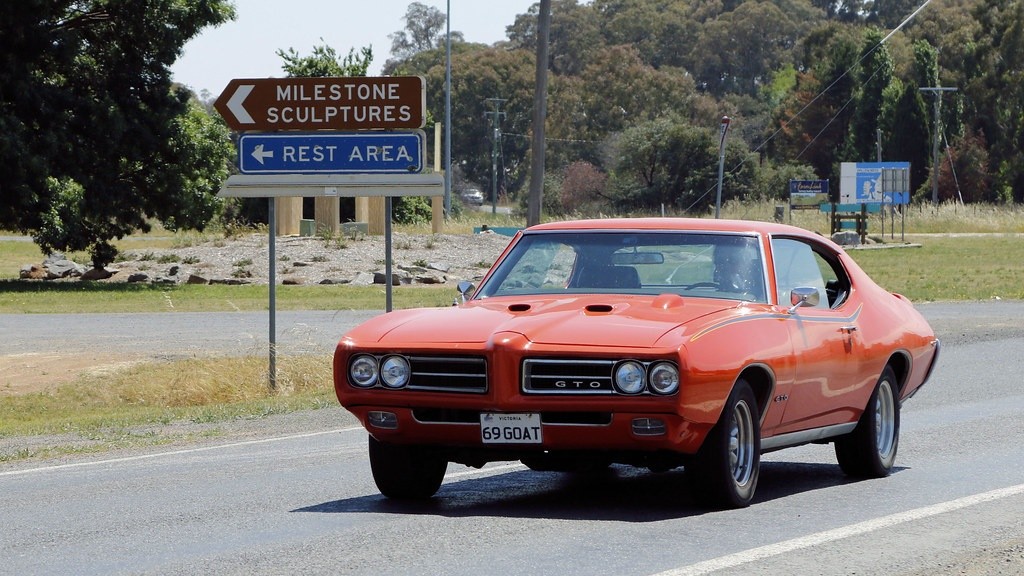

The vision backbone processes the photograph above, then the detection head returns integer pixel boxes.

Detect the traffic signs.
[239,129,428,177]
[211,72,427,133]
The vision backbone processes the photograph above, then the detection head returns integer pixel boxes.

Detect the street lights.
[713,114,731,220]
[918,86,960,206]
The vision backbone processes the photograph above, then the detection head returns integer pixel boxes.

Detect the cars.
[460,187,484,206]
[331,215,941,511]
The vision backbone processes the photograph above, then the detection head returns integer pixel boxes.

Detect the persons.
[713,238,757,297]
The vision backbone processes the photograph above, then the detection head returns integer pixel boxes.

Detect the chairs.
[577,267,641,289]
